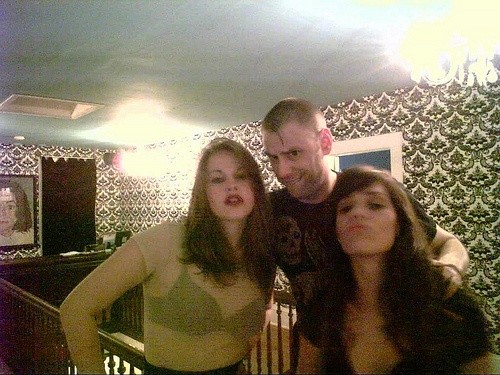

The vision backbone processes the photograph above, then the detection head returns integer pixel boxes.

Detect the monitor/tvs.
[114,231,132,247]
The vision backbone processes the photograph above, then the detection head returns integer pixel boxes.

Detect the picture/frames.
[0,174,38,249]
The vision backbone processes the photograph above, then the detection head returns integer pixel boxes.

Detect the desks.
[0,252,123,327]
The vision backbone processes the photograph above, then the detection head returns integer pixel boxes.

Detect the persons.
[261,98,470,375]
[296,165,490,375]
[59,137,273,375]
